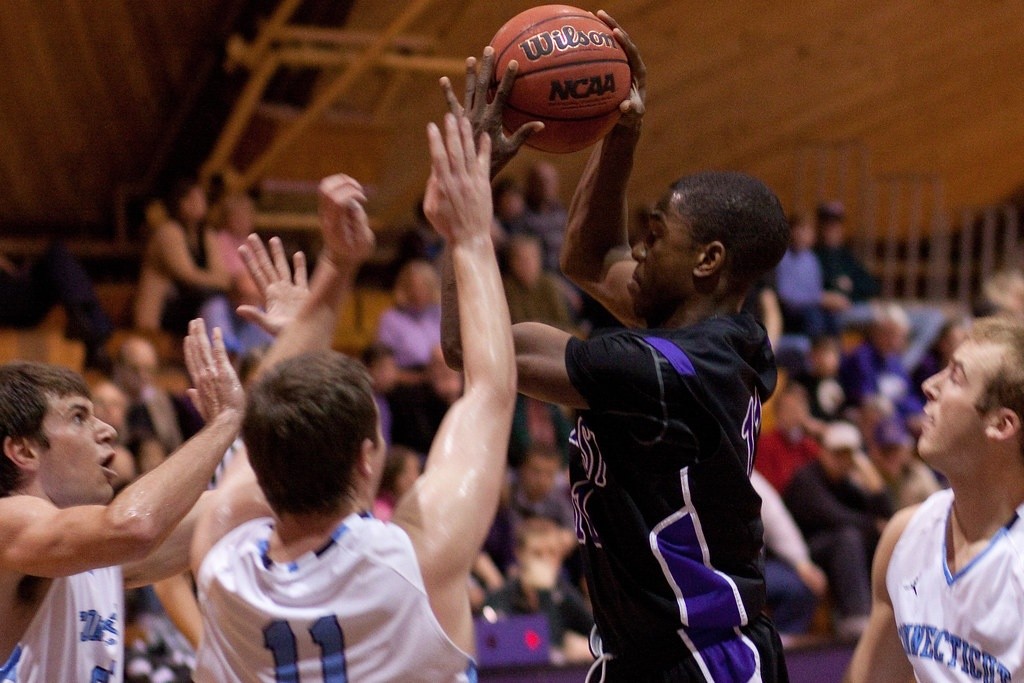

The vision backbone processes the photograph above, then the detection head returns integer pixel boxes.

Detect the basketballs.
[480,2,634,157]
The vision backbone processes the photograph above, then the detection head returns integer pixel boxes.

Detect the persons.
[439,9,792,683]
[841,321,1024,681]
[0,233,314,683]
[191,112,515,683]
[0,166,1023,662]
